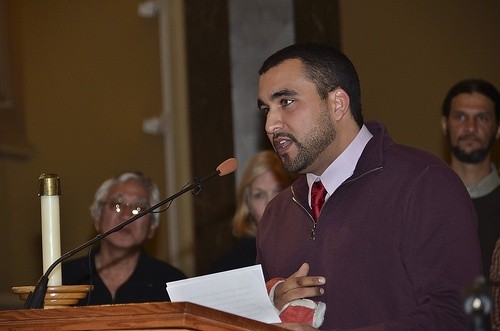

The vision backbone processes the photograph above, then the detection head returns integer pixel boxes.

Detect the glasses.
[101,200,149,216]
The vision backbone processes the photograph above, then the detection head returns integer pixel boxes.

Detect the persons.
[211,150,302,273]
[440,78,499,331]
[255,42,485,331]
[62,169,188,306]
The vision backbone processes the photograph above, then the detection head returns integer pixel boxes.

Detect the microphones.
[25,158,239,309]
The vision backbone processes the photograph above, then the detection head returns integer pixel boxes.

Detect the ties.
[311,181,327,223]
